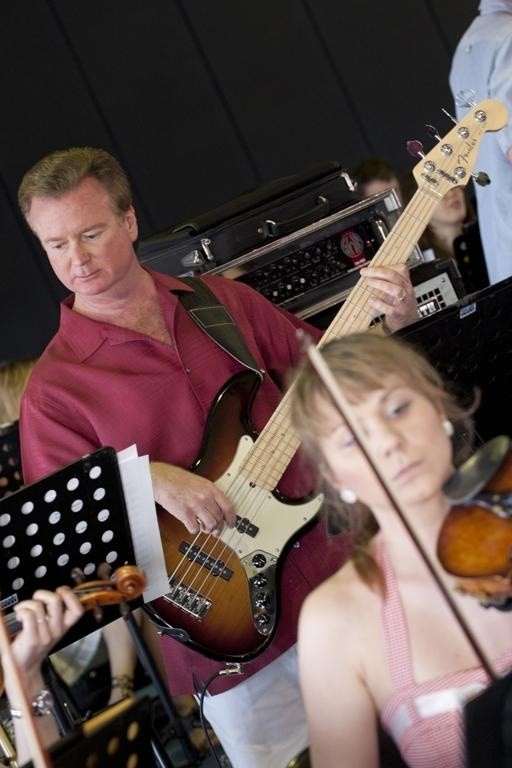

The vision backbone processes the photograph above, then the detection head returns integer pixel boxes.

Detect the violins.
[438,446,512,612]
[3,566,147,639]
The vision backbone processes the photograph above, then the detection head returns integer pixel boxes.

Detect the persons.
[289,331,512,768]
[0,586,85,768]
[447,0,512,285]
[404,166,467,260]
[346,152,403,206]
[15,146,419,768]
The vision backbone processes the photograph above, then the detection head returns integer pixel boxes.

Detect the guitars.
[141,90,508,663]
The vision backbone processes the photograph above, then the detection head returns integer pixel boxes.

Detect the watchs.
[9,688,55,718]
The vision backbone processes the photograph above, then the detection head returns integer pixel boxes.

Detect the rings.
[399,292,407,303]
[36,618,46,624]
